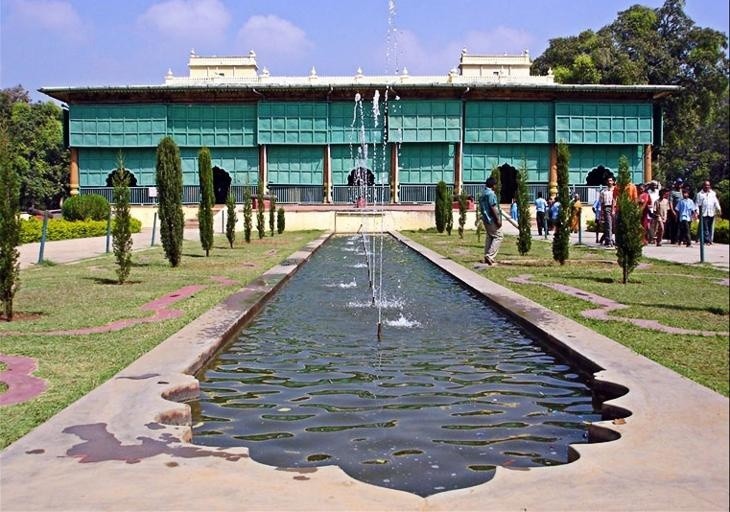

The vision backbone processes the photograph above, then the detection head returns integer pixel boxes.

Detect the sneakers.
[485,259,495,263]
[649,239,714,248]
[484,256,492,265]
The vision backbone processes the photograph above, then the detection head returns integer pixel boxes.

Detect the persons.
[534,173,723,247]
[511,198,518,220]
[478,176,503,266]
